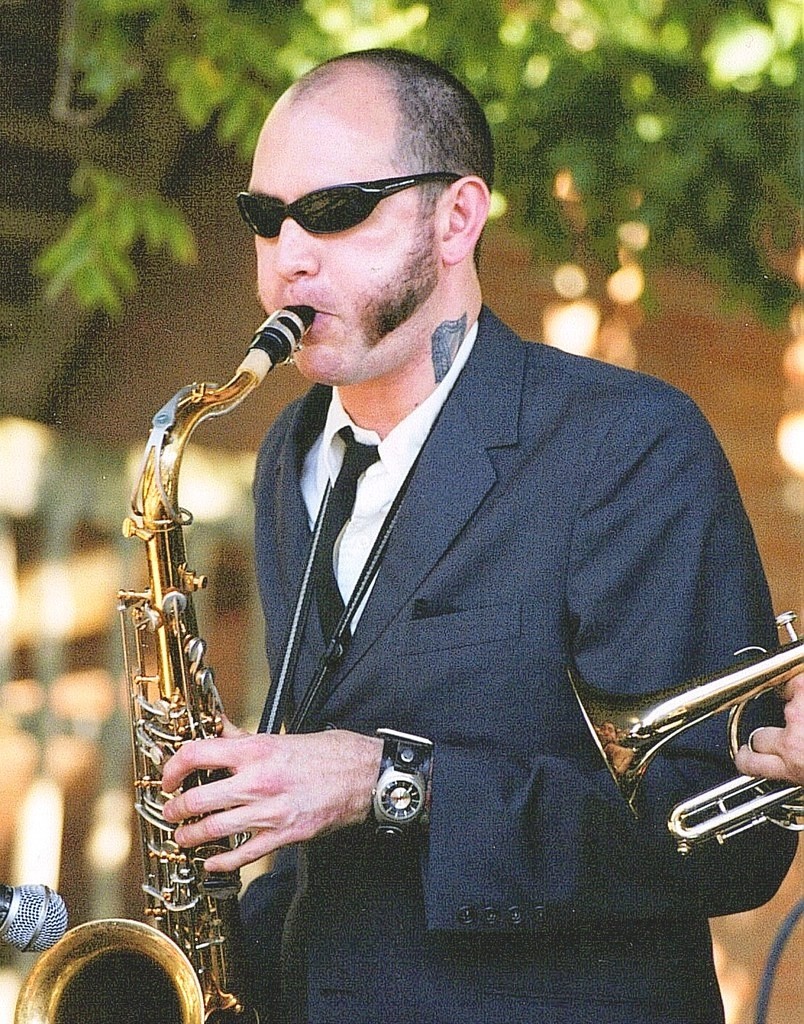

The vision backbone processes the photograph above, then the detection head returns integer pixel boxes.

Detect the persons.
[735,673,804,784]
[167,46,799,1023]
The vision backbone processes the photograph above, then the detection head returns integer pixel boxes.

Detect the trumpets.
[563,611,804,851]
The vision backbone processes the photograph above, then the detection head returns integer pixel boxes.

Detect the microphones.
[0,884,69,953]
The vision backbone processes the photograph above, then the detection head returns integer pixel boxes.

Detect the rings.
[747,726,764,752]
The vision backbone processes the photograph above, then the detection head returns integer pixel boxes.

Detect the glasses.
[236,172,463,238]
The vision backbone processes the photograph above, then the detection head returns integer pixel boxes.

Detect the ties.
[312,424,381,647]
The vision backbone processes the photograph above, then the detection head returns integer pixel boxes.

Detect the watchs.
[366,724,433,851]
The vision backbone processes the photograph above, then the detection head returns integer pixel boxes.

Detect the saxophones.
[11,304,318,1024]
